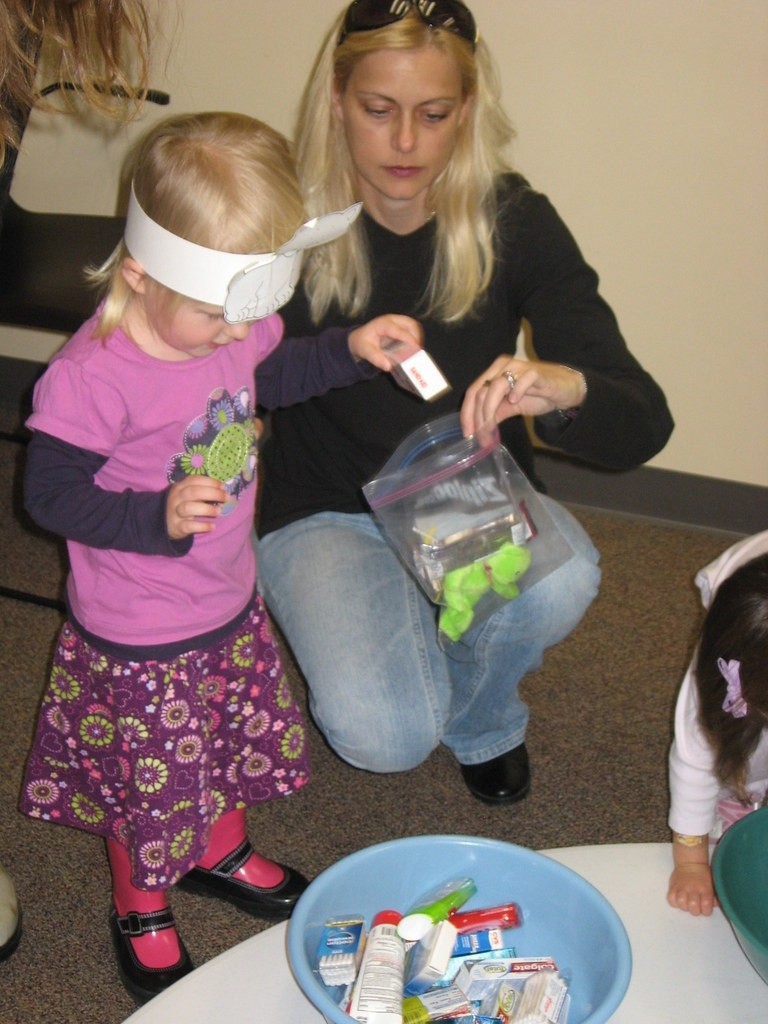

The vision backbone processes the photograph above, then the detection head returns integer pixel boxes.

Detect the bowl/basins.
[711,806,768,986]
[286,834,631,1024]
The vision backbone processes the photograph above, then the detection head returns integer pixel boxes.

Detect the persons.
[0,1,422,1007]
[667,528,768,917]
[256,0,675,807]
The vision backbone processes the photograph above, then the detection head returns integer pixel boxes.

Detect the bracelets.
[558,364,588,421]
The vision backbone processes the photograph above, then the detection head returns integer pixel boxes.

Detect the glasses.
[336,0,476,55]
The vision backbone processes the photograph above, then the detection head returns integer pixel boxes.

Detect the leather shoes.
[460,740,531,806]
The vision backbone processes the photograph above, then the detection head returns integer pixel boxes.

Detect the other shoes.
[173,839,310,922]
[0,862,23,962]
[108,897,194,1007]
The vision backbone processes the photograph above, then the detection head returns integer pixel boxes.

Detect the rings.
[502,371,519,391]
[482,380,492,388]
